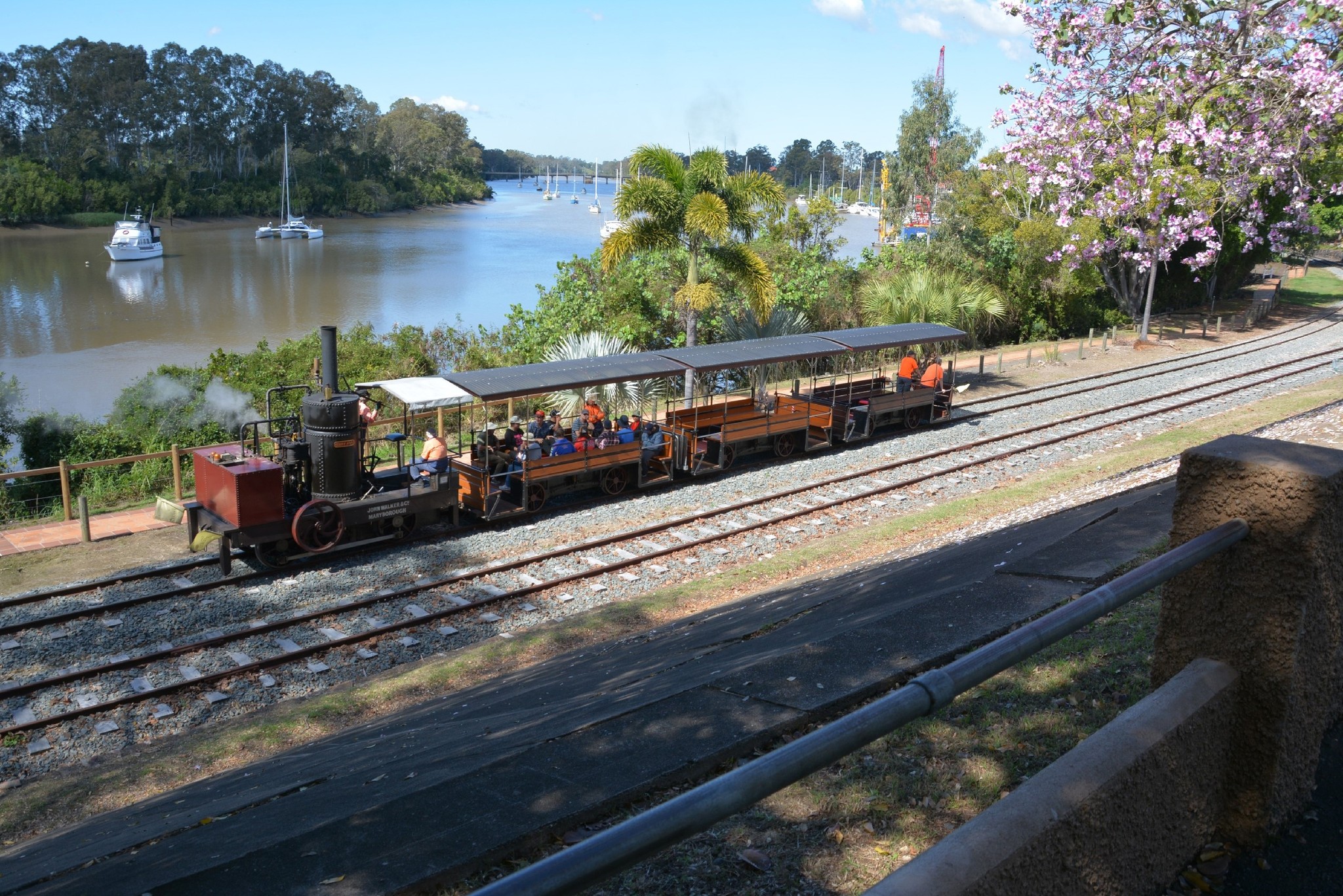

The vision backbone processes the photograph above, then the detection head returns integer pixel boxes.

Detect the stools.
[430,466,459,490]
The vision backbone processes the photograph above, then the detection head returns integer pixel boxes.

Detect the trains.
[149,323,967,576]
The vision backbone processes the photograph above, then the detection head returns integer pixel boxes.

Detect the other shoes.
[490,478,501,484]
[641,470,647,476]
[499,485,511,491]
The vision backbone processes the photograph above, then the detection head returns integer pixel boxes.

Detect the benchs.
[508,441,641,480]
[665,398,766,429]
[448,458,501,516]
[772,397,833,431]
[853,388,933,415]
[813,377,894,403]
[617,417,675,467]
[643,420,705,458]
[896,375,953,405]
[800,393,856,424]
[470,423,590,472]
[705,411,809,447]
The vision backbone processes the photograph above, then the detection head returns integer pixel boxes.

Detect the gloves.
[375,400,382,410]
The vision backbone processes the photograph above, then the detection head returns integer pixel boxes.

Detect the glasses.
[632,415,639,418]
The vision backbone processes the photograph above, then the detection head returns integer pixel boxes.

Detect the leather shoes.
[410,480,424,488]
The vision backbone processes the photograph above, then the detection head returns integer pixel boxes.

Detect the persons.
[615,415,634,444]
[499,433,542,491]
[584,394,605,437]
[408,427,447,488]
[549,428,577,456]
[640,422,663,477]
[547,409,562,436]
[526,411,555,456]
[630,409,644,441]
[571,409,595,444]
[477,422,510,484]
[573,426,596,452]
[356,389,381,460]
[504,415,525,460]
[593,419,620,450]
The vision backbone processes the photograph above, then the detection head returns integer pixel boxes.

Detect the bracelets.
[520,450,523,451]
[587,420,591,423]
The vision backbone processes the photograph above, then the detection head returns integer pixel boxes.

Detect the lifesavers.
[123,230,129,235]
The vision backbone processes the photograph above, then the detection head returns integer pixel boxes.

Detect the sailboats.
[517,167,523,187]
[533,165,550,194]
[253,124,324,240]
[580,168,587,194]
[570,166,579,204]
[588,157,601,213]
[599,169,632,241]
[794,147,948,247]
[551,163,560,198]
[543,173,552,200]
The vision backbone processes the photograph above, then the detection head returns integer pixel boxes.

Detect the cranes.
[908,44,947,227]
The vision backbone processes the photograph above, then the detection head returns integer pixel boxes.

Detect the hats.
[632,410,642,417]
[907,350,917,356]
[549,409,560,417]
[934,359,942,363]
[582,409,589,414]
[642,423,654,433]
[536,411,545,416]
[357,389,372,397]
[604,420,612,428]
[482,422,498,432]
[426,428,436,435]
[521,432,535,441]
[510,415,523,423]
[588,394,597,402]
[617,415,630,428]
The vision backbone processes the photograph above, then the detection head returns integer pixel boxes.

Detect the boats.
[103,202,164,261]
